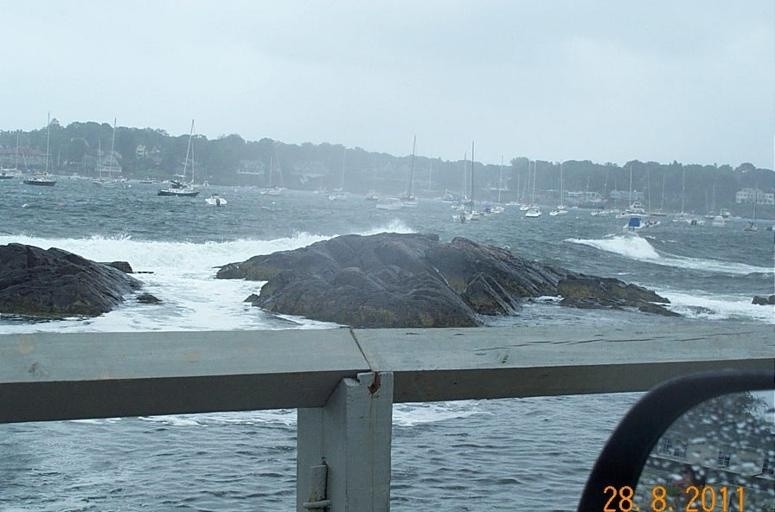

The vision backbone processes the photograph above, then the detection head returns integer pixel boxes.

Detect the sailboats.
[310,129,421,211]
[1,130,22,180]
[434,141,586,222]
[72,107,289,206]
[22,110,57,187]
[587,163,775,235]
